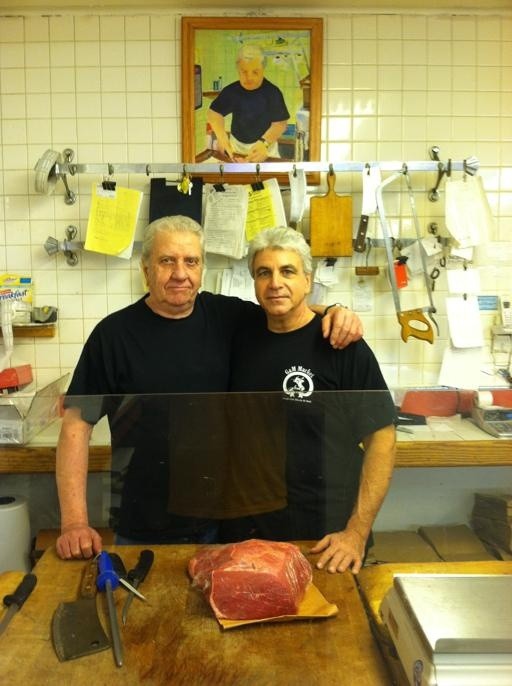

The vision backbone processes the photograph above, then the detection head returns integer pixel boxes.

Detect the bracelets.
[323,303,350,314]
[259,137,270,149]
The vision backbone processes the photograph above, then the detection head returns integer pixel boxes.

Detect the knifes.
[0,573,37,638]
[355,164,382,253]
[51,560,112,663]
[288,167,307,232]
[107,553,148,601]
[122,550,155,625]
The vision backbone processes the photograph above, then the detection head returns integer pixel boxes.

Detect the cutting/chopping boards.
[356,560,512,640]
[0,539,396,686]
[309,170,353,257]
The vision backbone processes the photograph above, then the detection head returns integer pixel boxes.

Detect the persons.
[214,225,399,578]
[205,43,292,165]
[50,214,368,565]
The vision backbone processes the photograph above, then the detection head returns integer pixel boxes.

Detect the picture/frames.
[180,15,321,187]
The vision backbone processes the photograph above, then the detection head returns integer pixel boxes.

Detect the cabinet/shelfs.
[45,148,480,275]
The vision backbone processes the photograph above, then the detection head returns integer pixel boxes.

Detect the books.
[242,178,289,241]
[82,181,144,262]
[149,177,205,225]
[199,182,248,260]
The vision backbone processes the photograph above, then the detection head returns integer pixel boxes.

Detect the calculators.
[472,407,512,438]
